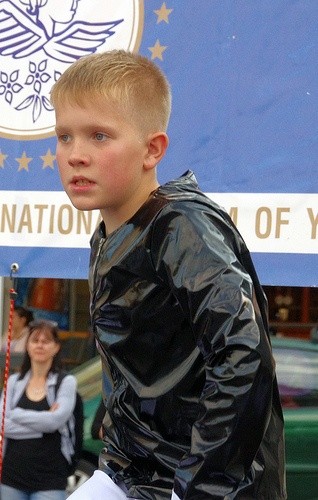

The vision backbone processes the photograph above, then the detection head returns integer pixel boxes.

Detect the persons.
[0,320,76,500]
[3,307,33,355]
[50,50,287,500]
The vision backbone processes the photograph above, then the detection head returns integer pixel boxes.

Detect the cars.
[70,334,317,500]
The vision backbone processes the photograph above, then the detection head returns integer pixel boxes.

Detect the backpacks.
[54,375,85,475]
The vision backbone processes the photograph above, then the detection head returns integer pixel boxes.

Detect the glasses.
[28,320,58,327]
